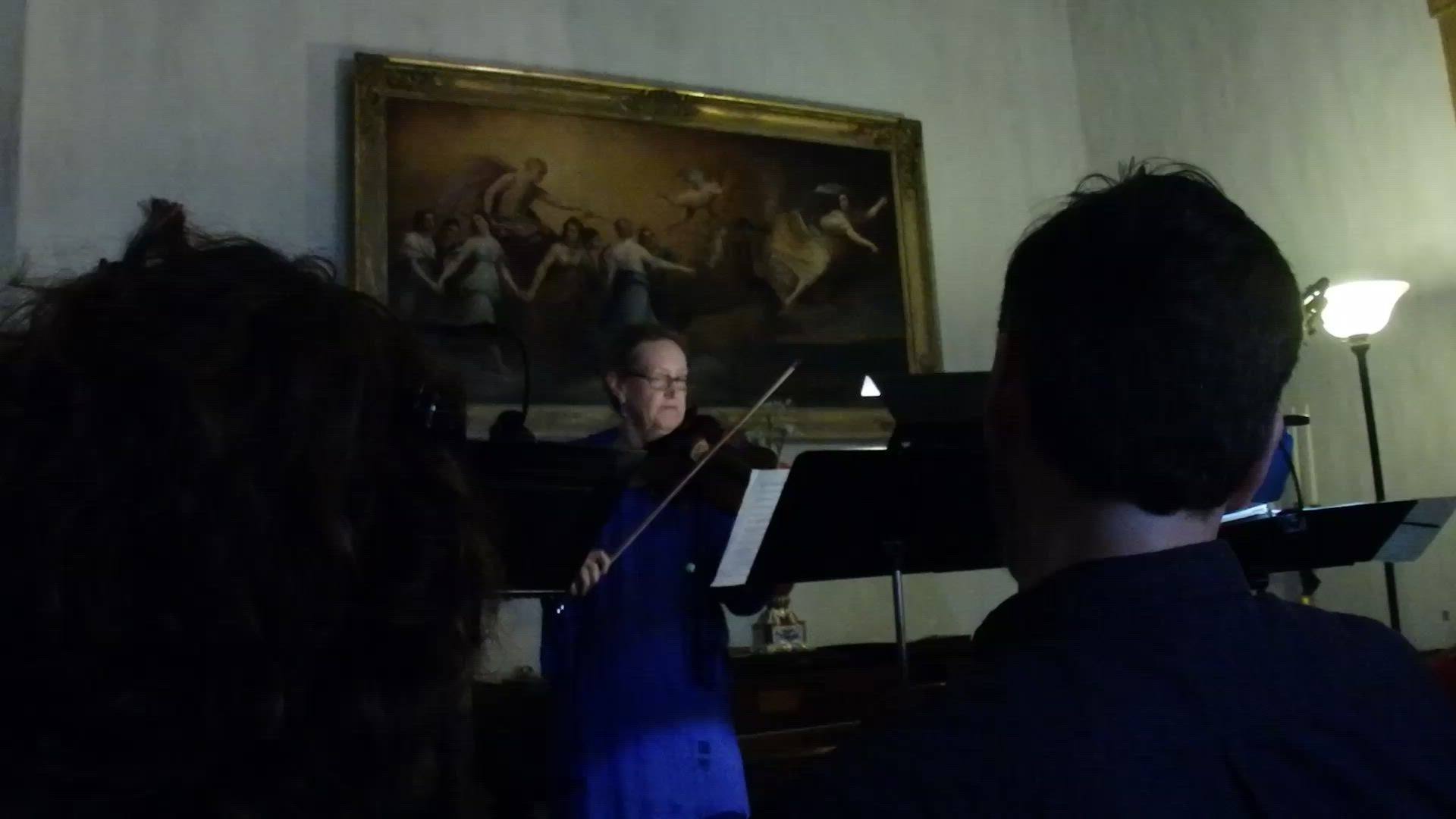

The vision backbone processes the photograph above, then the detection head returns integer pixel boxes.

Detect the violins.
[658,415,779,499]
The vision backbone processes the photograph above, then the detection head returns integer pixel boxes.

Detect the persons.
[782,156,1456,819]
[540,332,788,819]
[0,200,503,819]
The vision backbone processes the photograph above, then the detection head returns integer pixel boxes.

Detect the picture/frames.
[352,53,944,445]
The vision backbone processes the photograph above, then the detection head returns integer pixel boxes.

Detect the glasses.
[620,370,689,392]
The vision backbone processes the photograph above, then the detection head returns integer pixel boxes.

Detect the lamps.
[1302,280,1410,635]
